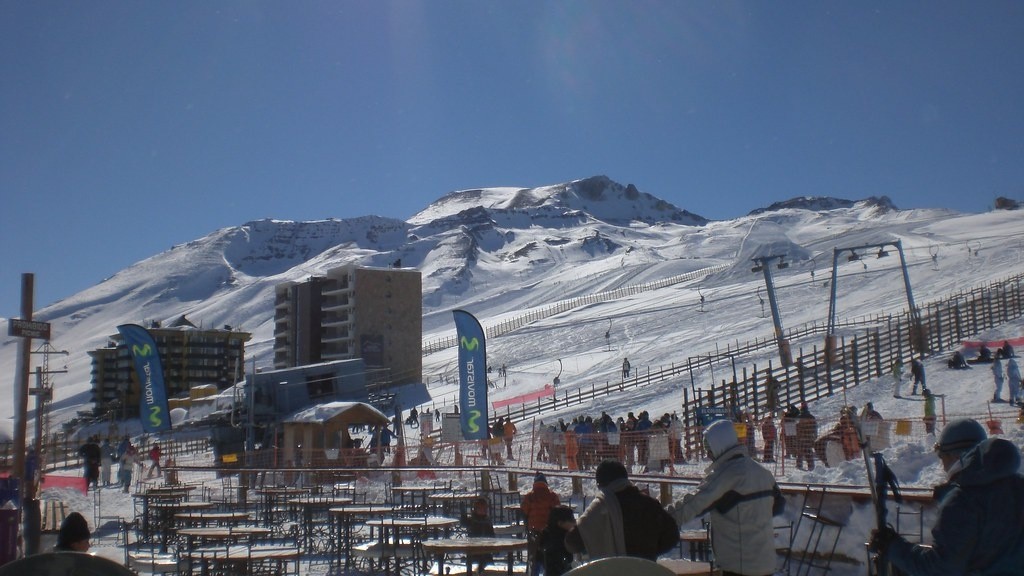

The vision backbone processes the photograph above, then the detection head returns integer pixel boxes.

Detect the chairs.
[93,457,794,576]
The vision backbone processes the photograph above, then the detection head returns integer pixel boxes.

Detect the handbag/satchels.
[608,433,620,445]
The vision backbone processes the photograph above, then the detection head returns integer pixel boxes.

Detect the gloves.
[867,526,899,555]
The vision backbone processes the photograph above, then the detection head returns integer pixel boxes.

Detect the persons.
[738,370,882,471]
[893,358,926,398]
[623,358,630,378]
[868,418,1024,576]
[80,434,163,494]
[663,419,785,576]
[520,472,576,576]
[947,341,1014,369]
[558,459,679,563]
[992,355,1004,402]
[1007,359,1022,406]
[351,405,459,453]
[460,499,497,576]
[51,512,90,552]
[482,411,688,474]
[488,363,506,377]
[921,388,936,436]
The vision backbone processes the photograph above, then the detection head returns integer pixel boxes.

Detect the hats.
[596,458,628,486]
[57,512,91,544]
[936,418,987,457]
[534,473,546,482]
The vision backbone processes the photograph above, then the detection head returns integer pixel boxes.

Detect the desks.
[174,545,305,576]
[172,510,249,525]
[328,507,405,566]
[502,502,579,563]
[679,528,710,563]
[365,517,460,575]
[254,488,310,500]
[421,537,530,575]
[656,557,722,576]
[392,485,451,516]
[428,492,486,518]
[131,491,188,521]
[175,525,270,551]
[145,501,213,553]
[289,496,352,553]
[144,485,196,500]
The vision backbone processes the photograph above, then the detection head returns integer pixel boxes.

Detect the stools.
[782,485,847,576]
[896,504,925,545]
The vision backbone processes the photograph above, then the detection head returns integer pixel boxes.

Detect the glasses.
[934,442,940,451]
